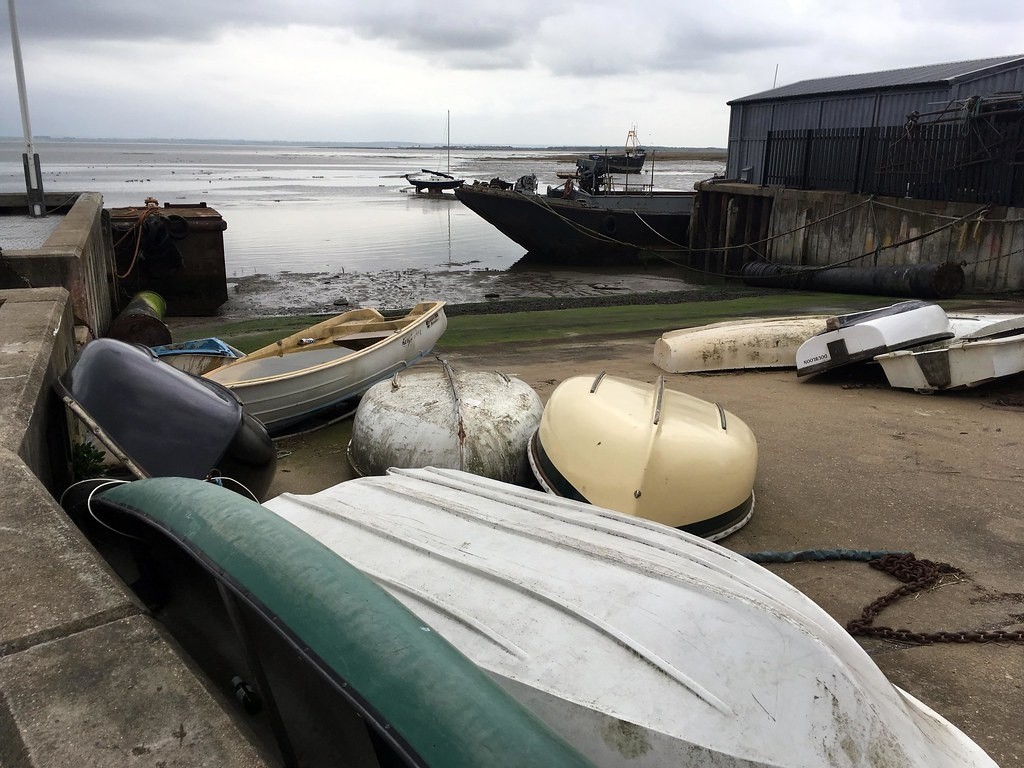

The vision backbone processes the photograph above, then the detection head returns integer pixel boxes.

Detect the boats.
[54,336,279,508]
[72,472,587,766]
[794,298,951,378]
[529,370,758,542]
[253,464,1002,766]
[457,126,694,265]
[200,301,446,436]
[401,111,466,189]
[337,358,548,490]
[871,333,1024,394]
[650,313,849,375]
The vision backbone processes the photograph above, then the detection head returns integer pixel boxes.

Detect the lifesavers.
[133,214,173,249]
[166,213,190,240]
